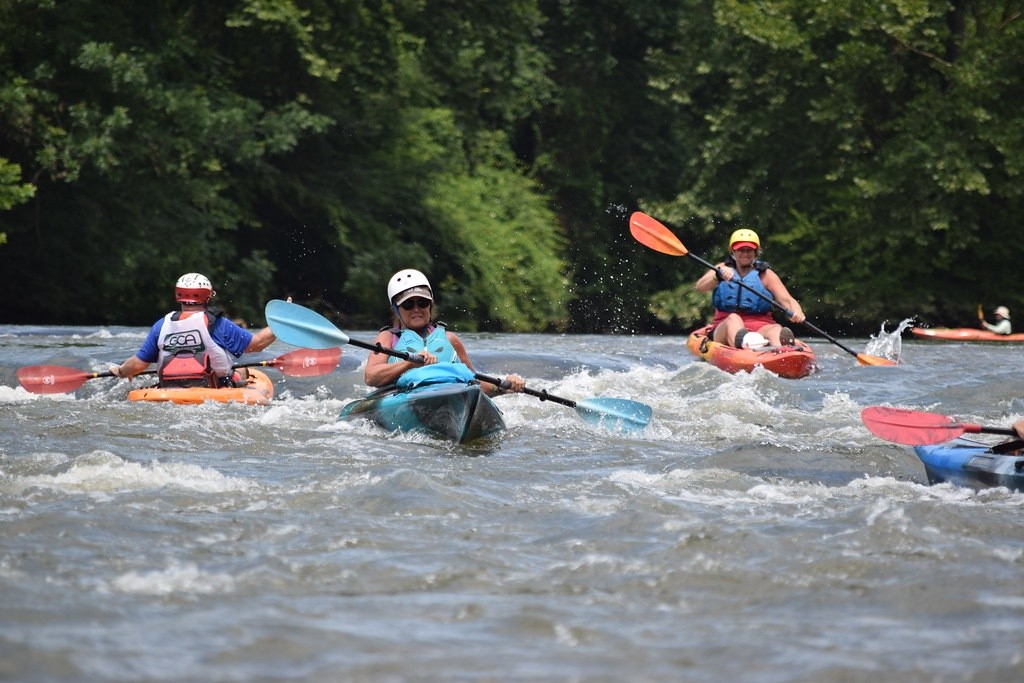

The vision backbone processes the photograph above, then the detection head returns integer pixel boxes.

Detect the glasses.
[402,297,430,310]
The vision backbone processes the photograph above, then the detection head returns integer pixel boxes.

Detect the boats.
[911,326,1024,347]
[128,366,274,407]
[689,323,817,378]
[369,381,507,444]
[917,437,1024,497]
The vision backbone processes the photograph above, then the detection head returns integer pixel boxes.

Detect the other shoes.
[779,327,795,346]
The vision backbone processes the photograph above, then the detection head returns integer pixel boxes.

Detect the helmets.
[729,229,760,254]
[387,269,433,307]
[175,273,213,304]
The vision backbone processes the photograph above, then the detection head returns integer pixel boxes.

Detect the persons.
[695,229,806,349]
[364,269,526,398]
[109,273,292,388]
[981,305,1012,335]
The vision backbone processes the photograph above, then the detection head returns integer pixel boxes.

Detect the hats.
[394,285,434,306]
[994,306,1011,319]
[733,242,757,250]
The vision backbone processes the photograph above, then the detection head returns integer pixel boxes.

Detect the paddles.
[860,406,1019,446]
[978,304,986,331]
[629,209,899,368]
[15,346,342,395]
[265,299,651,436]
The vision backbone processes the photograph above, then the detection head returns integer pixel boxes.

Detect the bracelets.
[118,368,122,374]
[492,385,498,396]
